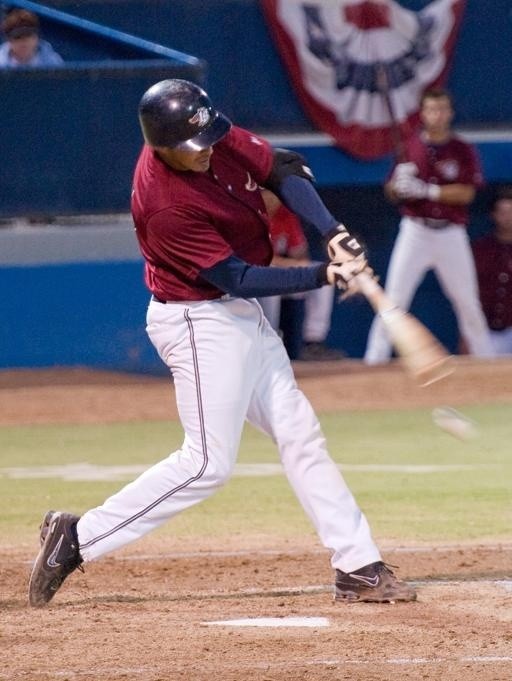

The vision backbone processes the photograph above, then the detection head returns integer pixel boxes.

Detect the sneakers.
[334,560,416,603]
[29,510,83,606]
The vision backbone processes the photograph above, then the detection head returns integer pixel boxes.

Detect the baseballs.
[434,411,472,439]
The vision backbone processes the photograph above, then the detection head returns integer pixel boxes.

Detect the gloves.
[393,161,441,200]
[321,221,380,294]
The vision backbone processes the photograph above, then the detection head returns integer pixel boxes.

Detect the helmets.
[138,77,232,151]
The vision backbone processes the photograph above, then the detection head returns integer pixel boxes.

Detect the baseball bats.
[355,270,459,394]
[372,64,407,167]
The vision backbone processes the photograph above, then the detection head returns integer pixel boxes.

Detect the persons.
[363,84,496,367]
[260,186,348,359]
[27,79,417,609]
[1,8,63,69]
[456,196,512,356]
